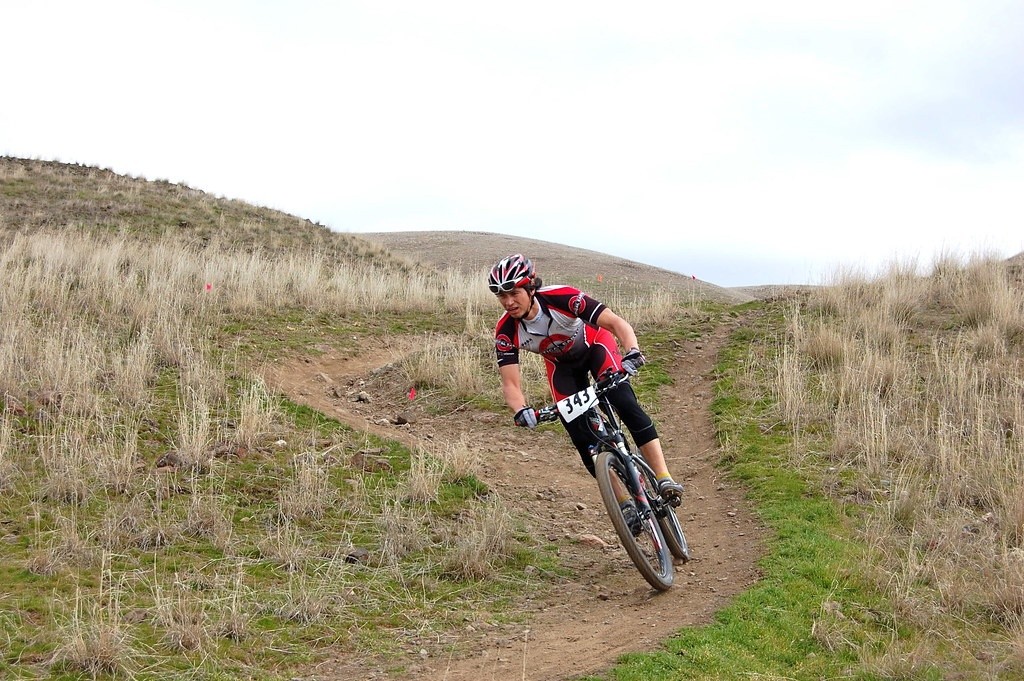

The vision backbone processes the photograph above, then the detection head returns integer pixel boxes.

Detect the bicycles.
[514,357,690,591]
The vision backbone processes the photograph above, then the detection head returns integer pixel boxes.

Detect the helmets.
[488,253,536,292]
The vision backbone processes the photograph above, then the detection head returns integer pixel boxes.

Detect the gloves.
[513,407,539,428]
[621,348,646,375]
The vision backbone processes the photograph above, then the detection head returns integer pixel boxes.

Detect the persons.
[488,252,685,535]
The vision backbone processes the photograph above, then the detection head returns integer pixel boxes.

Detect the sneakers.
[620,500,637,527]
[658,479,683,499]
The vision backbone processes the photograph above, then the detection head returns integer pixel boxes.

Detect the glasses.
[489,276,523,294]
